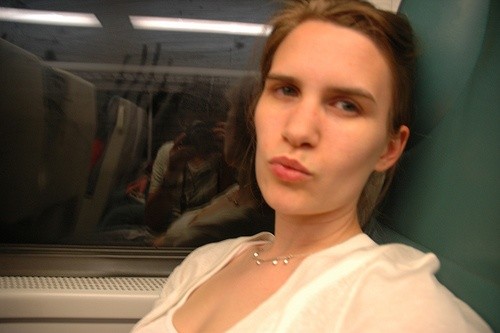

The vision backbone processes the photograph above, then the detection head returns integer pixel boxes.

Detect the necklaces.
[253,241,311,265]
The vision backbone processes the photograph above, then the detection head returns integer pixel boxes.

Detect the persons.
[153,75,273,247]
[131,0,495,333]
[108,81,236,232]
